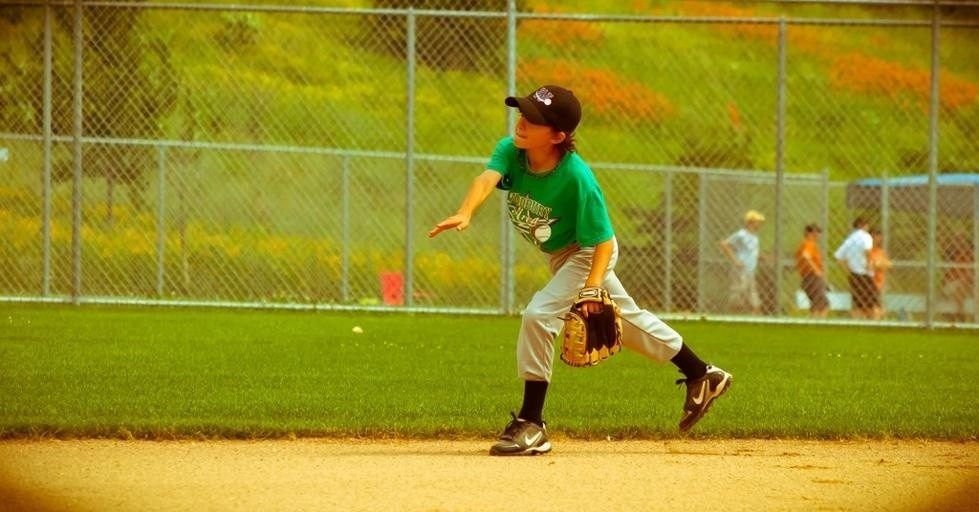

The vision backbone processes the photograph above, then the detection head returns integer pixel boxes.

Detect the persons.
[834,218,882,320]
[429,83,734,456]
[721,210,766,315]
[870,228,892,319]
[936,222,976,323]
[797,223,832,318]
[758,253,780,314]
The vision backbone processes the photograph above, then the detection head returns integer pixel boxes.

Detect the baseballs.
[352,326,364,333]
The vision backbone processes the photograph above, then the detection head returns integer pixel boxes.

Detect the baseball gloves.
[556,286,623,367]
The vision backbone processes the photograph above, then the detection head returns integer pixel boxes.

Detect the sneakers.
[676,363,733,431]
[490,411,551,455]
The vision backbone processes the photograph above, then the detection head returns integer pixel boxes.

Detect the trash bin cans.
[381,271,403,307]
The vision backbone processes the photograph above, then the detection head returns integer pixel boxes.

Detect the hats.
[744,210,766,224]
[504,85,581,133]
